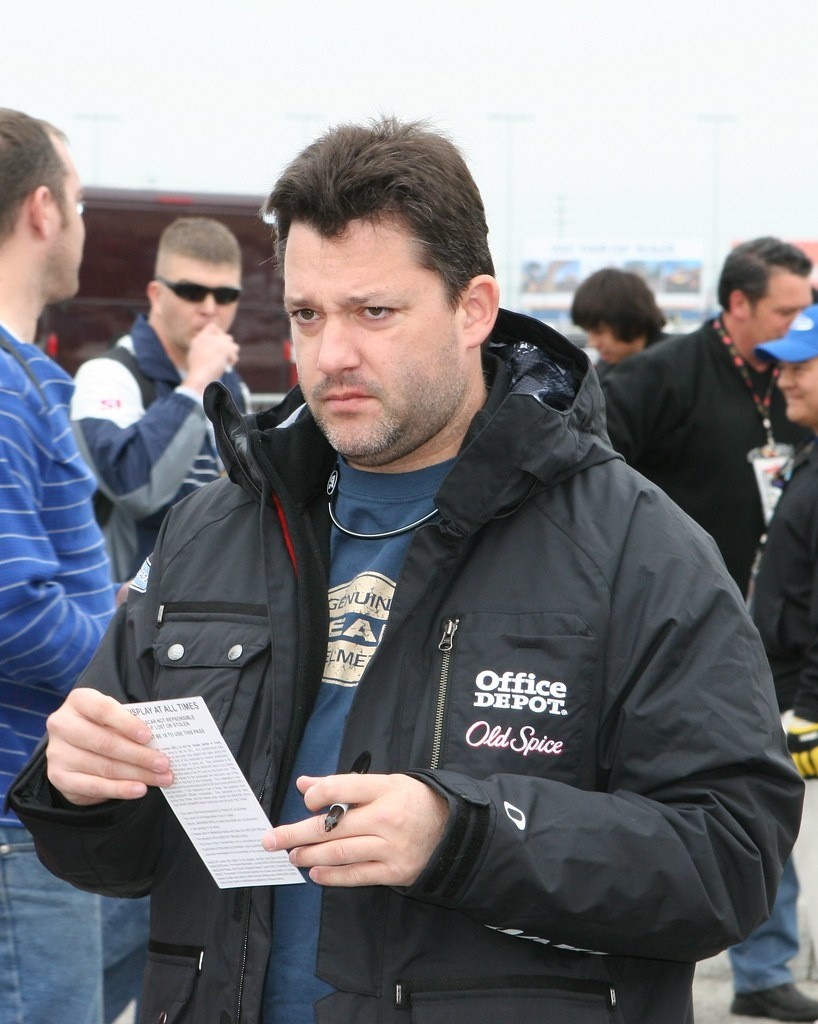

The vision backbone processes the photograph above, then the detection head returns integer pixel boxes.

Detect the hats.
[753,303,818,362]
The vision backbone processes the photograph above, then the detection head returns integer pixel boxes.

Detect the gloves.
[786,713,818,780]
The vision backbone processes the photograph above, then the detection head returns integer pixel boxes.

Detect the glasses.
[154,275,243,303]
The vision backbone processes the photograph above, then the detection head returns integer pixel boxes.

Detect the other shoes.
[730,983,818,1022]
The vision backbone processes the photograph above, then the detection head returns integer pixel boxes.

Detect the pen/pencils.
[324,750,372,833]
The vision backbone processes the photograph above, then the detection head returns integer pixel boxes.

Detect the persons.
[0,106,153,1024]
[73,215,257,585]
[600,237,818,1024]
[746,303,818,723]
[565,269,685,383]
[6,119,806,1024]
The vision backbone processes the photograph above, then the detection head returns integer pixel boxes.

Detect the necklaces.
[324,464,439,539]
